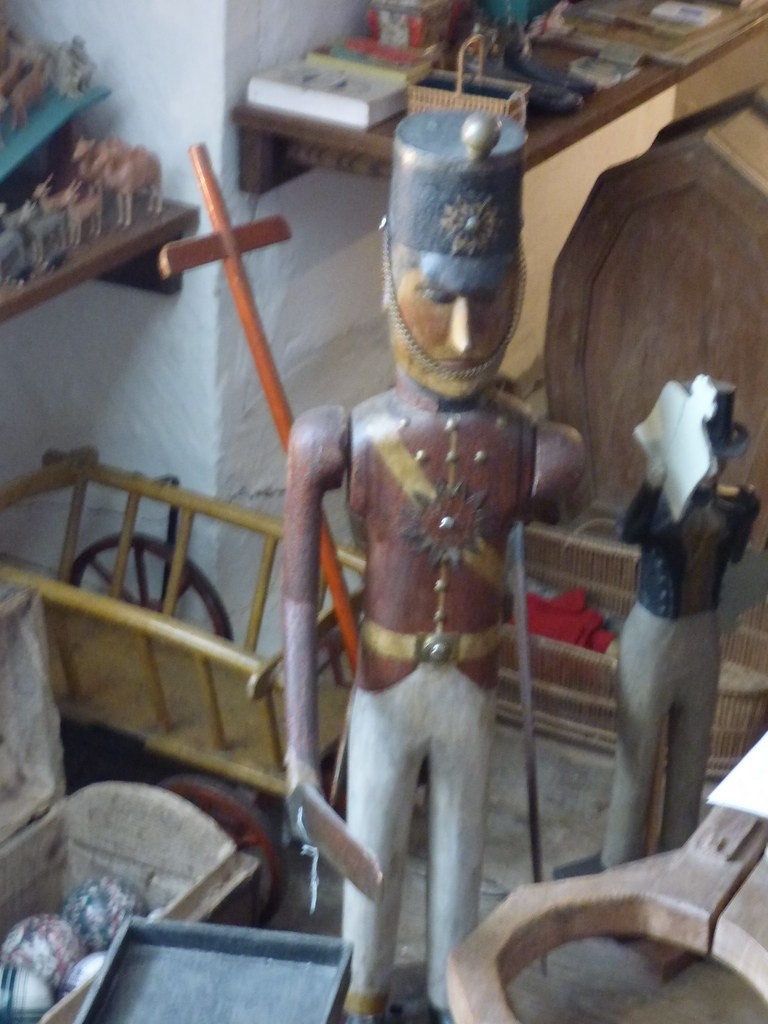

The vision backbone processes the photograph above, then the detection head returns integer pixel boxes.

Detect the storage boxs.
[1,780,264,1024]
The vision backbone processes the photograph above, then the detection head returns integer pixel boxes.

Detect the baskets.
[406,34,531,126]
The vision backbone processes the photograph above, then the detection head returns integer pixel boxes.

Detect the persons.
[281,110,586,1024]
[601,374,761,868]
